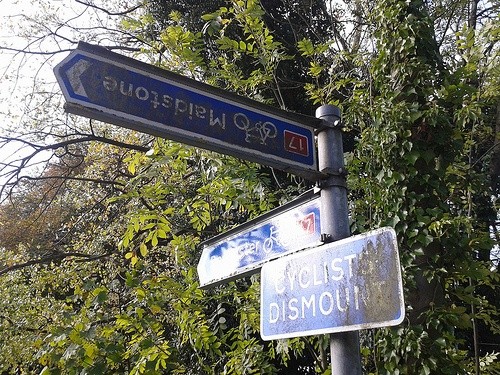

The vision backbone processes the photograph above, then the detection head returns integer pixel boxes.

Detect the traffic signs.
[49,42,318,181]
[189,186,323,292]
[257,225,406,341]
